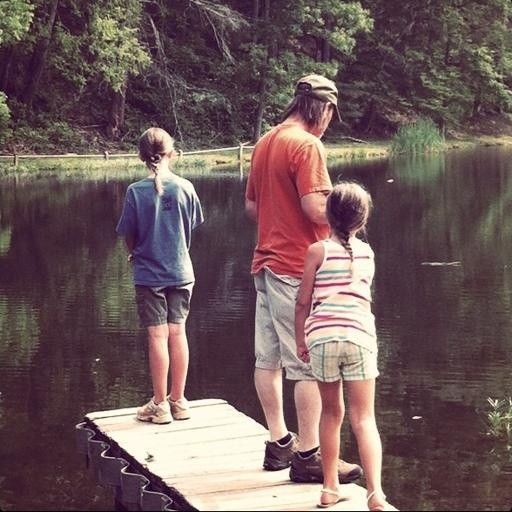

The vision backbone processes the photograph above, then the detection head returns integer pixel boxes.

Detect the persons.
[294,182,396,511]
[244,73,365,485]
[114,127,206,427]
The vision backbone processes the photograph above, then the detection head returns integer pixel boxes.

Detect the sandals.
[367,490,387,512]
[316,488,349,508]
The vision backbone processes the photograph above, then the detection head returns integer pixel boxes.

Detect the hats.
[295,73,343,124]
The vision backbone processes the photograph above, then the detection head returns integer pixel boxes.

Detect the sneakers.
[289,447,364,484]
[136,395,173,424]
[167,394,191,420]
[263,432,300,471]
